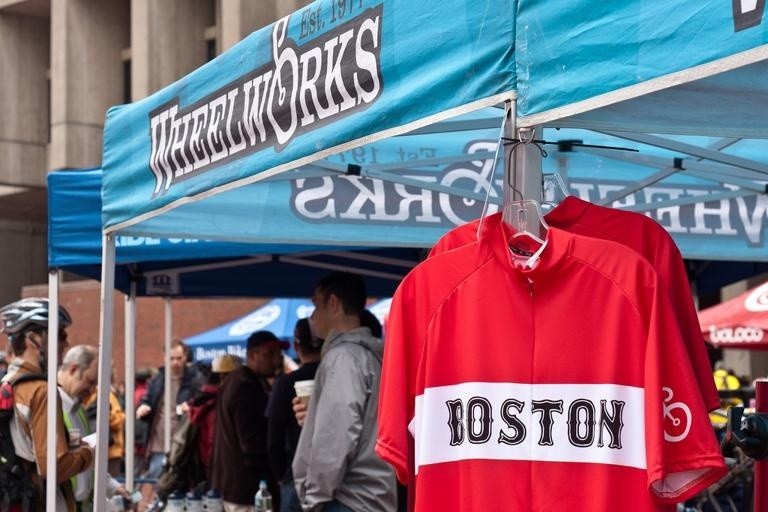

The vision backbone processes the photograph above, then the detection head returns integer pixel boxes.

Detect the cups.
[175,404,184,417]
[68,429,81,451]
[294,378,314,414]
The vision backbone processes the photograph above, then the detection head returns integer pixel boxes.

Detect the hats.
[246,331,291,351]
[211,355,242,374]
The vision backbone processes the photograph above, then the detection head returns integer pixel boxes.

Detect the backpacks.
[153,391,217,504]
[0,375,50,512]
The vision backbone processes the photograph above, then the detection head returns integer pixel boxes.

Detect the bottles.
[254,480,272,512]
[166,488,223,512]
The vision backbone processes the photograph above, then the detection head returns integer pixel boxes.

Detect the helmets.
[0,297,72,336]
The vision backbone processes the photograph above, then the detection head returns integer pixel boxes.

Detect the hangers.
[497,135,572,267]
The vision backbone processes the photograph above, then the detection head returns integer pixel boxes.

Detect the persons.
[0,270,400,512]
